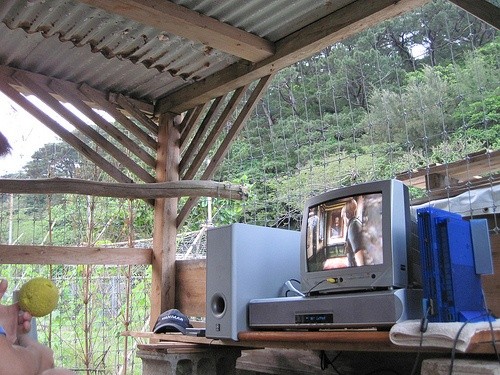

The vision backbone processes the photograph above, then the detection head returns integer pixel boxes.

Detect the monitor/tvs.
[300,179,422,294]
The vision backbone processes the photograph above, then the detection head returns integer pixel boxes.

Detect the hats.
[151,308,192,335]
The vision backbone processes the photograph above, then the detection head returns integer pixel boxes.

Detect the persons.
[341,199,372,266]
[332,217,341,236]
[0,279,74,375]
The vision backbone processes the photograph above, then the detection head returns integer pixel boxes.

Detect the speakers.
[205,222,301,341]
